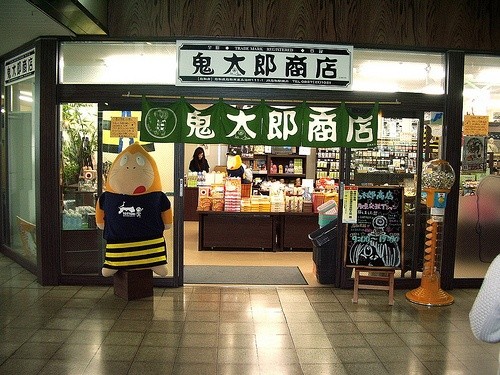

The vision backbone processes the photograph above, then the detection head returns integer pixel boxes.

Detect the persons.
[468,253,500,343]
[189,147,210,173]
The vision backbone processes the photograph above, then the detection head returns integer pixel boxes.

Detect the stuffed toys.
[224,148,247,184]
[95,141,171,278]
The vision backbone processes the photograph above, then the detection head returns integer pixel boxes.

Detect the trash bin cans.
[308,219,338,283]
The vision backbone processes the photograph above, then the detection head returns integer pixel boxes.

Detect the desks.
[196,211,281,252]
[183,187,199,221]
[280,212,320,252]
[63,191,96,210]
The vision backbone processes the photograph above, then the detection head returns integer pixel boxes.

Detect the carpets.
[183,265,309,285]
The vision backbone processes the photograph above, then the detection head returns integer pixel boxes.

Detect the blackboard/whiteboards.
[343,186,406,270]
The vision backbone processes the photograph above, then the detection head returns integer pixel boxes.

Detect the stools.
[353,268,395,306]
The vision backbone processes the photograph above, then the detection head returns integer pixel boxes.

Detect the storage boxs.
[471,168,490,181]
[197,178,338,212]
[459,174,475,186]
[294,169,303,174]
[294,159,302,169]
[86,212,97,228]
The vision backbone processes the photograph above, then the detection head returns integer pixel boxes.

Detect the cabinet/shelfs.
[242,153,307,197]
[423,128,440,153]
[486,152,494,174]
[315,142,417,191]
